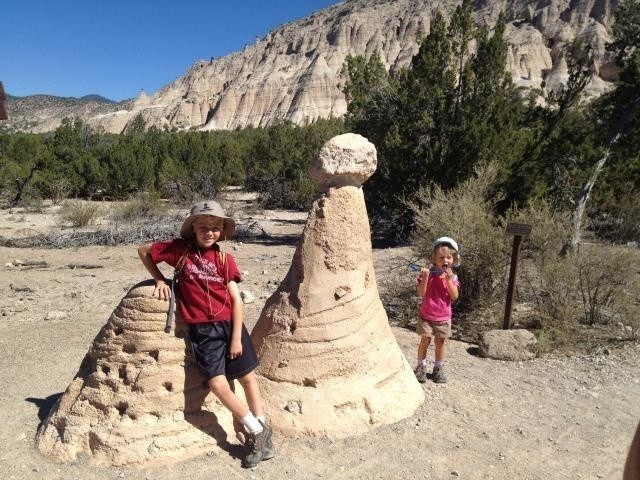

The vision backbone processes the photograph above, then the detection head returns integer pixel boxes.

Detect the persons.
[415,237,462,383]
[137,201,275,468]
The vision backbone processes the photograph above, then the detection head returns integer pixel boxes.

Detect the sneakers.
[413,365,428,383]
[431,365,447,383]
[240,417,275,468]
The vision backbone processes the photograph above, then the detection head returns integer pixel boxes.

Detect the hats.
[179,200,236,241]
[431,236,459,251]
